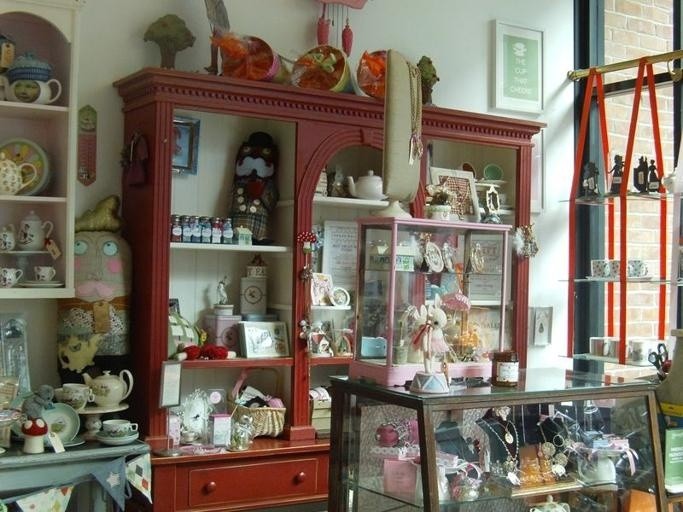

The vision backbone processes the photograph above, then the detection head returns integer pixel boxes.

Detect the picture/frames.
[430,166,482,222]
[491,19,546,115]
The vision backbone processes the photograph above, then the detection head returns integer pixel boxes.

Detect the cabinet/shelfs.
[328,367,670,512]
[348,217,513,387]
[0,0,82,300]
[111,66,547,512]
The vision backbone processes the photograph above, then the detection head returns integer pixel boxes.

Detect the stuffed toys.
[231,130,276,240]
[400,291,470,395]
[57,198,131,381]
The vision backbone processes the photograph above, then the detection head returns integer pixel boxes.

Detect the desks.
[0,440,151,512]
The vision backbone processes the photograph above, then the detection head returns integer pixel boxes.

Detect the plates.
[43,402,81,444]
[46,439,86,449]
[95,432,140,444]
[75,403,130,416]
[19,279,64,289]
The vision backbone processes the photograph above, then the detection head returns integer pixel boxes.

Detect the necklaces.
[408,62,424,161]
[442,177,471,221]
[546,423,569,446]
[481,418,519,475]
[493,418,513,444]
[444,433,468,463]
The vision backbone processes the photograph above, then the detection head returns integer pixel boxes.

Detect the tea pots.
[0,151,43,195]
[346,168,391,202]
[10,209,56,251]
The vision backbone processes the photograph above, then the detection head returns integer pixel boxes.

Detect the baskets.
[227,367,286,439]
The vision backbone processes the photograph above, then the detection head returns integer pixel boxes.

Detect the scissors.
[648,343,669,378]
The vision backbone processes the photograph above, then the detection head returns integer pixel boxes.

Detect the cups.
[590,258,607,278]
[621,258,650,278]
[0,266,24,289]
[31,264,58,283]
[63,383,97,411]
[608,260,627,277]
[589,334,657,364]
[81,369,135,408]
[102,419,140,439]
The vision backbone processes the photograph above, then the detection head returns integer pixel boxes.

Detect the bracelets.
[388,419,413,446]
